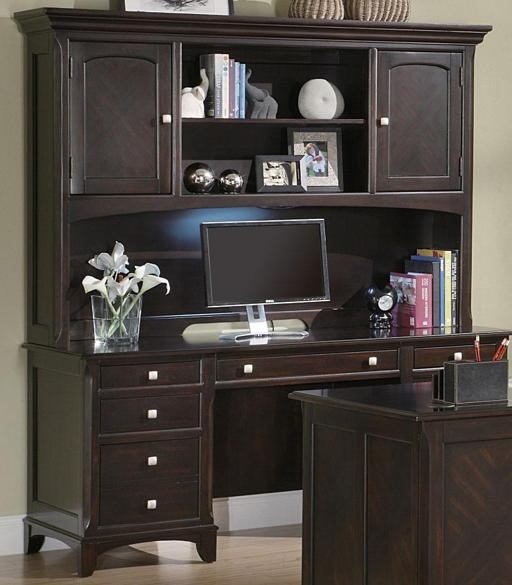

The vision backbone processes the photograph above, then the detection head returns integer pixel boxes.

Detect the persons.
[388,247,459,330]
[197,52,247,121]
[303,148,314,176]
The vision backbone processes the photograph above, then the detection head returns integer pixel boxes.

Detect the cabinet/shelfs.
[9,8,493,576]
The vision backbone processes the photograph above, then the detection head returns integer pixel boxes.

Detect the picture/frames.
[254,126,344,194]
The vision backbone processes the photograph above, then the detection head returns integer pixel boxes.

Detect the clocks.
[365,282,398,326]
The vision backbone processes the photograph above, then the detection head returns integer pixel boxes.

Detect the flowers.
[81,240,171,335]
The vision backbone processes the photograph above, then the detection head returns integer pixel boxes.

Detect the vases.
[91,295,143,347]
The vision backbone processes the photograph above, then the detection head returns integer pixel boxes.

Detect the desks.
[286,383,510,585]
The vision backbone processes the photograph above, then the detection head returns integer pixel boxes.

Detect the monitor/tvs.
[199,218,331,341]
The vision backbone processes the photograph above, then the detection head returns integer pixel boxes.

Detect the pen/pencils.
[474,335,510,362]
